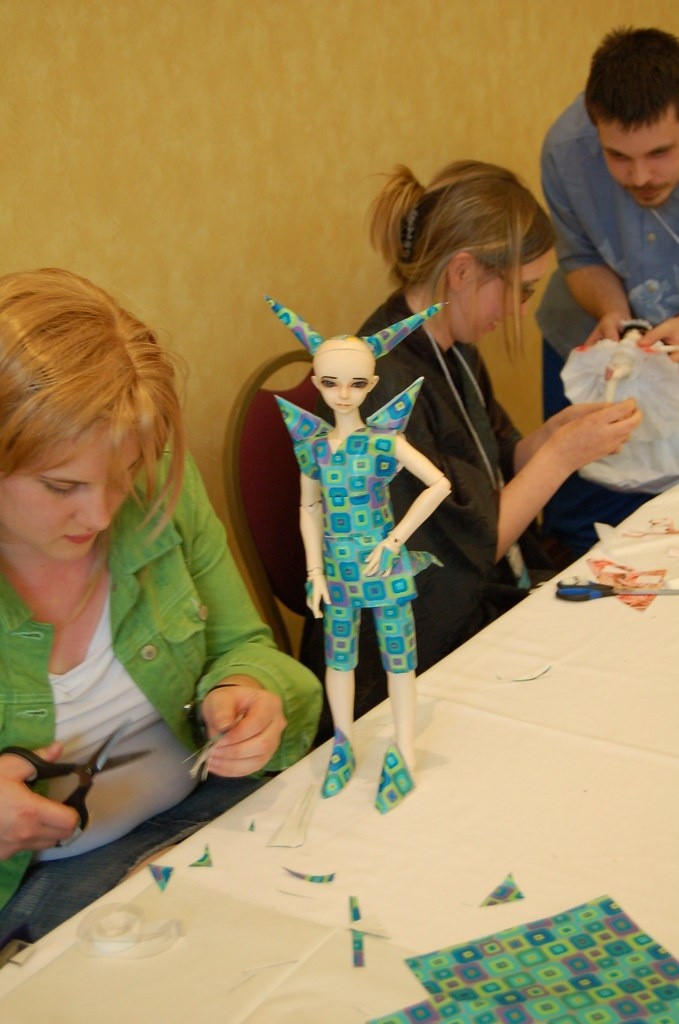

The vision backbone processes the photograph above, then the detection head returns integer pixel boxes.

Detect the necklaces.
[51,582,98,631]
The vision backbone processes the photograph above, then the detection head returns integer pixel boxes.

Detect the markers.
[645,345,678,353]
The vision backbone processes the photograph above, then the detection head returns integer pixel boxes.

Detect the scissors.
[556,574,679,603]
[2,714,158,847]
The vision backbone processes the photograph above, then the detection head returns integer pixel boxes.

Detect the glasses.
[475,257,536,305]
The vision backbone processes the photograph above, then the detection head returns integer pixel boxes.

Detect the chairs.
[222,350,321,658]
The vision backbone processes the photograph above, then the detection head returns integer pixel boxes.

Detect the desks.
[0,479,678,1024]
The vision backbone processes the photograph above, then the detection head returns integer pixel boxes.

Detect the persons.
[268,158,643,729]
[265,295,454,815]
[534,28,679,527]
[0,267,323,941]
[591,317,679,407]
